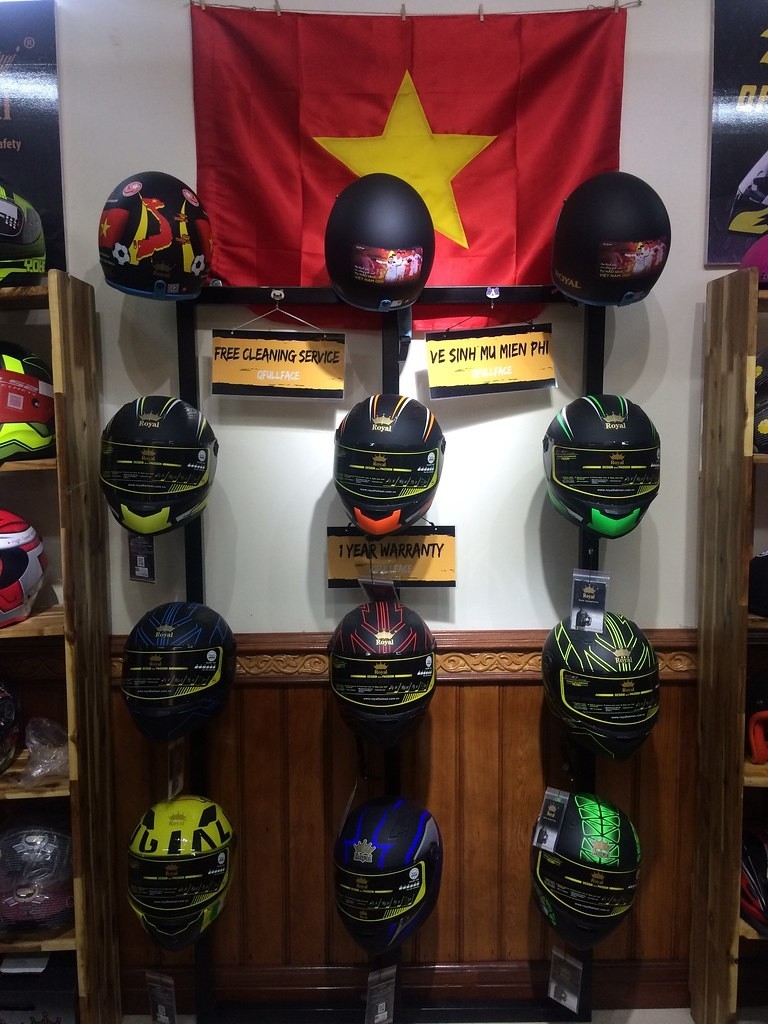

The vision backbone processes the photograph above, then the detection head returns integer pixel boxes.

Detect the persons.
[360,250,375,270]
[536,826,548,848]
[575,606,590,631]
[373,250,422,282]
[554,984,567,1003]
[606,240,666,274]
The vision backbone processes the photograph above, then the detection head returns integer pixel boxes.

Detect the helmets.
[0,186,47,289]
[531,789,640,948]
[543,393,660,539]
[0,509,48,629]
[552,170,671,304]
[0,826,73,937]
[332,798,442,953]
[333,393,446,539]
[98,395,218,535]
[0,682,22,771]
[326,174,435,311]
[126,795,237,949]
[0,340,54,462]
[541,612,660,760]
[100,170,212,300]
[118,601,237,742]
[328,600,436,729]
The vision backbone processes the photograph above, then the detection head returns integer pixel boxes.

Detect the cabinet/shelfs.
[688,269,768,1024]
[0,270,121,1024]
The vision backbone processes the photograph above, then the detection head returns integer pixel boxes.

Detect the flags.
[190,0,627,331]
